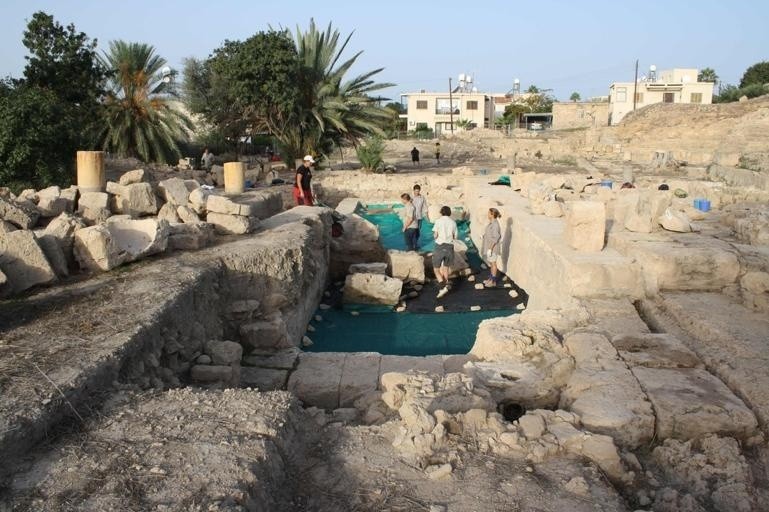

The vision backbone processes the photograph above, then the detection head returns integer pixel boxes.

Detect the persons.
[409,184,430,239]
[400,193,419,251]
[481,208,501,287]
[293,155,316,206]
[432,206,458,298]
[201,148,215,172]
[435,143,441,164]
[411,147,419,166]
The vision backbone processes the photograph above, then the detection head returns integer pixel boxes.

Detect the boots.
[483,278,496,287]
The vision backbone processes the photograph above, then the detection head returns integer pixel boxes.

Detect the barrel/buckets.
[602,180,612,190]
[694,198,711,212]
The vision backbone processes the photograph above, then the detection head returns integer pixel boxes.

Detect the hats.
[303,155,315,163]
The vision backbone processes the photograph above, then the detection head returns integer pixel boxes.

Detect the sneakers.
[436,284,452,298]
